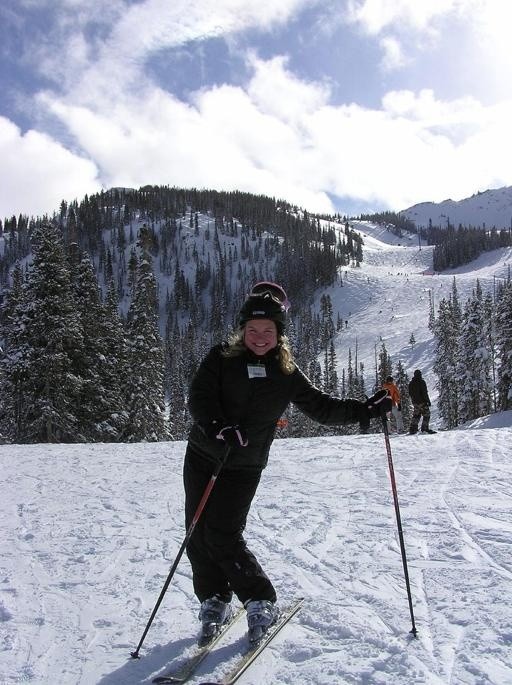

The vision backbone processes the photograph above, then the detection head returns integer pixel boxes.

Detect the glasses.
[249,282,287,305]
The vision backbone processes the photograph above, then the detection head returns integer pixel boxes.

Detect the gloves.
[364,389,393,420]
[214,422,248,448]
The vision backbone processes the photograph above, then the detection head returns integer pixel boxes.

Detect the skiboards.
[153,596,304,685]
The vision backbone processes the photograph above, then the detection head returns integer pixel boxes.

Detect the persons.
[407,369,435,435]
[381,375,408,436]
[182,280,394,640]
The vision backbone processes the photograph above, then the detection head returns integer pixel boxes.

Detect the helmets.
[238,299,287,328]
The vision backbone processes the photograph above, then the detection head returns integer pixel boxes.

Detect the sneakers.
[245,599,279,629]
[199,594,228,627]
